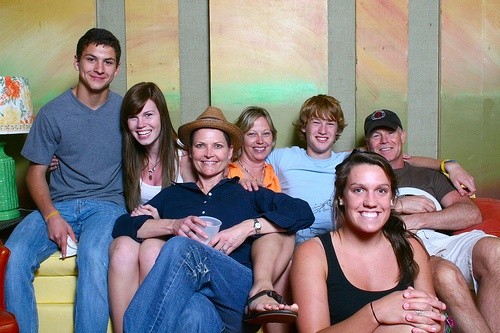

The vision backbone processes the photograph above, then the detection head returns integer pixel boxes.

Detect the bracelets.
[441,159,457,173]
[370,301,381,325]
[44,210,60,222]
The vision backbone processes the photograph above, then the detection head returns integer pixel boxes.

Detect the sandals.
[243,289,298,322]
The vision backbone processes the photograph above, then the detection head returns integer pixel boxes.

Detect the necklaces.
[145,156,169,181]
[237,157,267,183]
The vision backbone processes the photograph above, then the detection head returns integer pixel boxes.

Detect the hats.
[364,110,402,136]
[178,106,243,153]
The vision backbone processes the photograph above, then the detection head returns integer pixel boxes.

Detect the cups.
[196,216,222,245]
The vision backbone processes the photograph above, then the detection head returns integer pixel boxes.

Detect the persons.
[4,27,132,333]
[47,82,198,333]
[112,106,316,333]
[227,107,300,326]
[337,109,500,333]
[230,94,476,333]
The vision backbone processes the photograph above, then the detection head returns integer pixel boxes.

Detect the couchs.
[33,197,500,333]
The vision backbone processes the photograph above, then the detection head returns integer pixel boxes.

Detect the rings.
[184,229,192,235]
[219,246,227,254]
[415,309,424,314]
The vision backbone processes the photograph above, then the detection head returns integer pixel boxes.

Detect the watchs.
[253,218,262,235]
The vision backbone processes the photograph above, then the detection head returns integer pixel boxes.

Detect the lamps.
[0,75,35,221]
